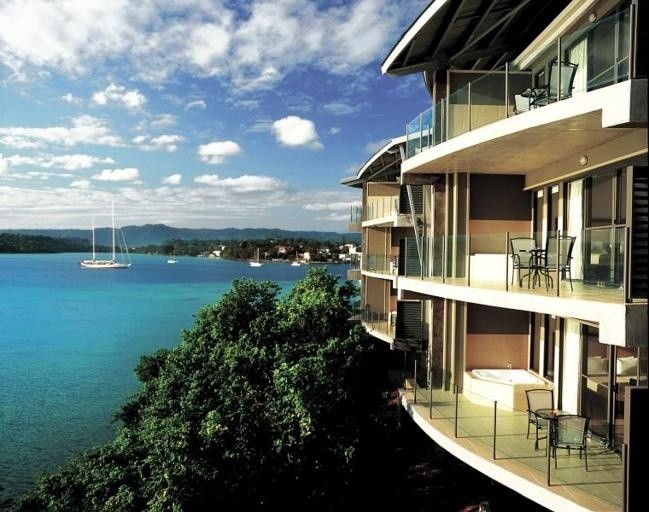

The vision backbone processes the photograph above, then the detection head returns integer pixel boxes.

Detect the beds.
[582,370,649,402]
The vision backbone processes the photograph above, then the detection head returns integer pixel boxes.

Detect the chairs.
[524,387,556,440]
[529,234,576,292]
[512,91,538,114]
[546,413,591,473]
[530,58,580,110]
[509,236,542,289]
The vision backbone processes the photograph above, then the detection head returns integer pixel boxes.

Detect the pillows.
[587,355,609,376]
[616,357,639,376]
[617,355,635,361]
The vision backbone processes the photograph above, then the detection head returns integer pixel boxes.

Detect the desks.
[520,85,551,109]
[519,248,554,290]
[531,406,571,459]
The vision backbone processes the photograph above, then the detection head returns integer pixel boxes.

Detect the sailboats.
[248,248,302,267]
[167,250,180,264]
[78,197,134,270]
